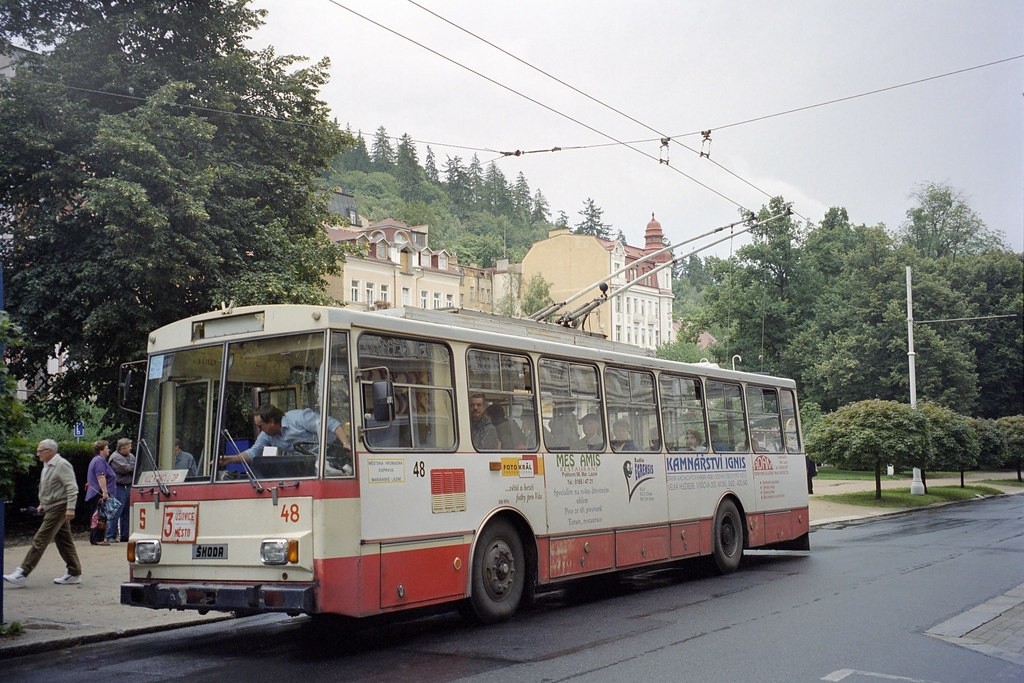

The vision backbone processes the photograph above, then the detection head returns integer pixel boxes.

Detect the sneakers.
[3,567,27,587]
[53,570,81,584]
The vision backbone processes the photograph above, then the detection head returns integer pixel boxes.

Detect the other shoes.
[92,541,110,546]
[107,539,119,543]
[120,539,128,542]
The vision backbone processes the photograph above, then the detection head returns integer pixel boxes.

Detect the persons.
[464,392,772,455]
[172,438,197,478]
[3,439,82,586]
[86,440,117,547]
[107,438,137,544]
[208,403,351,471]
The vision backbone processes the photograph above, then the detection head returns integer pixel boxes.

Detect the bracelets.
[343,442,347,444]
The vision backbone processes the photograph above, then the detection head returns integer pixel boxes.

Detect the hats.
[578,413,600,424]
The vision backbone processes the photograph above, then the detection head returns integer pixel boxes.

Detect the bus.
[116,299,811,622]
